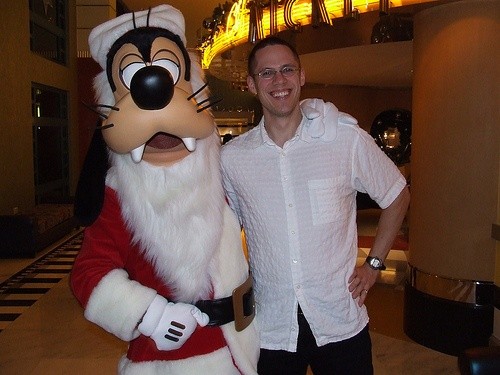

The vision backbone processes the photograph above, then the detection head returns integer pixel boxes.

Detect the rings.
[362,289,368,294]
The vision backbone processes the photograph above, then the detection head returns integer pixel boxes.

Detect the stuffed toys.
[58,3,357,375]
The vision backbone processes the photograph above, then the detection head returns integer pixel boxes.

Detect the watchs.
[366,256,387,272]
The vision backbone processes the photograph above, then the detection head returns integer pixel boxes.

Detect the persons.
[222,37,412,375]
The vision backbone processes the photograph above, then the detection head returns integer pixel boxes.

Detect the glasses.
[254,66,299,80]
